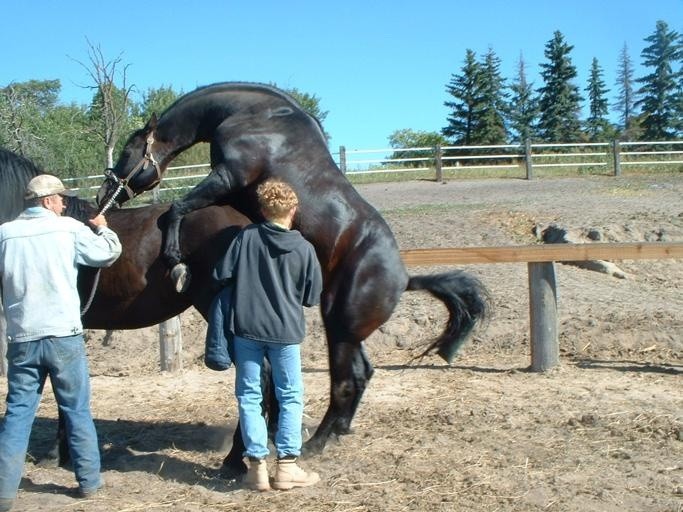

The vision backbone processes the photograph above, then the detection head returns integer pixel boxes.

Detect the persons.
[207,180,325,491]
[0,174,123,512]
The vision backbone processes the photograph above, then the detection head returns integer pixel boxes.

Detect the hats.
[25,174,77,200]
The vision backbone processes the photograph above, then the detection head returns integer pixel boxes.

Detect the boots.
[273,459,320,489]
[243,457,271,491]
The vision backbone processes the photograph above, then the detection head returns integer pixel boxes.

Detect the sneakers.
[81,479,103,497]
[0,497,12,511]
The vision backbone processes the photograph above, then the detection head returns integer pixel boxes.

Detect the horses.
[0,145,280,480]
[95,81,493,461]
